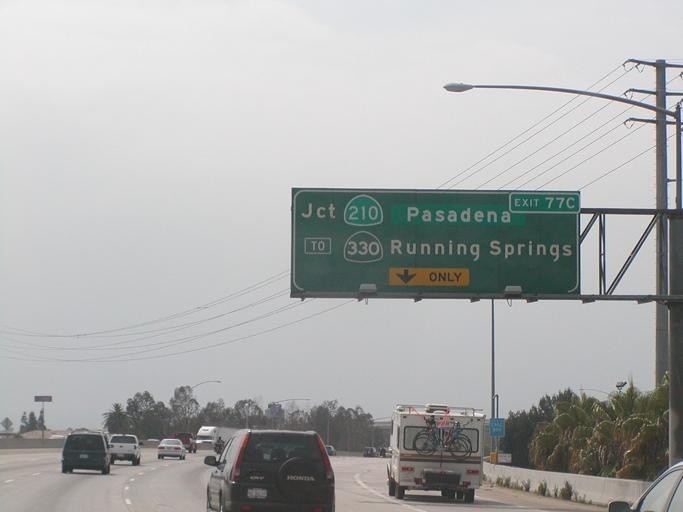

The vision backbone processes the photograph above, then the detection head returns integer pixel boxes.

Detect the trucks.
[197,427,233,443]
[387,404,486,502]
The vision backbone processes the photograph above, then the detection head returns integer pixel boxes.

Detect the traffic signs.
[291,187,581,301]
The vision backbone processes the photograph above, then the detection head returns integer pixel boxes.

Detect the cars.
[607,462,683,512]
[111,434,142,467]
[157,434,196,459]
[362,447,390,457]
[205,430,337,511]
[61,429,111,476]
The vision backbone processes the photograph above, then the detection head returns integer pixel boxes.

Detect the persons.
[214,437,224,452]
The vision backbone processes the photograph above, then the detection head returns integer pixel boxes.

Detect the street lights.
[444,82,682,462]
[186,378,220,388]
[272,398,309,429]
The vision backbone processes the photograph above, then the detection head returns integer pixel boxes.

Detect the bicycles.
[413,418,472,460]
